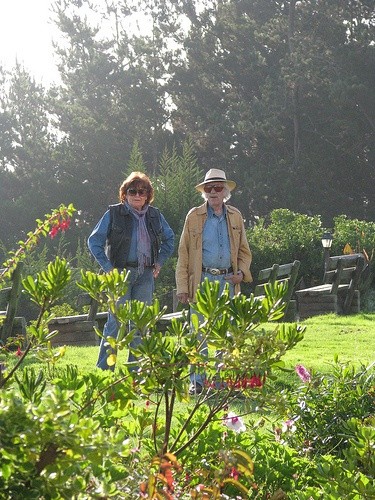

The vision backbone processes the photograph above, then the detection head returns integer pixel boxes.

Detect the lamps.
[321,233,334,248]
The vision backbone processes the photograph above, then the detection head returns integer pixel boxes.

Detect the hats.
[194,169,236,192]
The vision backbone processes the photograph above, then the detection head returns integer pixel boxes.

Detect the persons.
[176,168,253,396]
[87,171,175,379]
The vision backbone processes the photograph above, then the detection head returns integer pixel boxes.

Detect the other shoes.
[188,382,203,394]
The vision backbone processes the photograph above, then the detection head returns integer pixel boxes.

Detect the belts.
[127,262,154,268]
[202,266,233,275]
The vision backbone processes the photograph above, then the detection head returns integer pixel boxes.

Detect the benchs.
[0,261,27,348]
[47,268,109,347]
[295,253,366,318]
[246,260,301,322]
[156,288,189,337]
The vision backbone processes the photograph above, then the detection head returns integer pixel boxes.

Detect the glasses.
[127,189,147,196]
[204,185,224,193]
